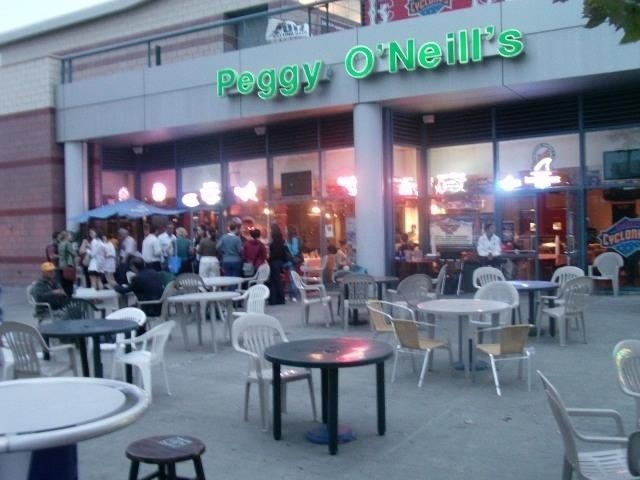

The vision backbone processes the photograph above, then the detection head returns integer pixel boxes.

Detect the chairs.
[0,320,83,380]
[465,322,534,399]
[425,263,448,300]
[343,274,379,331]
[534,276,595,347]
[534,369,639,480]
[173,272,211,352]
[331,270,360,316]
[611,339,640,428]
[53,300,106,352]
[0,320,53,380]
[113,319,177,403]
[470,266,521,325]
[388,320,455,389]
[85,307,148,381]
[134,280,177,343]
[288,272,334,328]
[221,284,270,344]
[389,275,433,329]
[468,282,520,345]
[362,297,415,374]
[231,313,317,432]
[588,252,625,298]
[536,267,583,331]
[298,254,329,284]
[233,261,268,312]
[21,282,71,344]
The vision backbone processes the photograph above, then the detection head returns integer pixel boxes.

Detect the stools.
[124,435,207,480]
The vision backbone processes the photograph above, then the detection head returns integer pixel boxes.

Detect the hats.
[41,262,57,271]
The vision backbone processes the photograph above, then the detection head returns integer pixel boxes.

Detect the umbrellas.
[67,199,192,222]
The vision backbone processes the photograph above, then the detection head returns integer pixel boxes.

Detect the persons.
[323,240,353,283]
[396,232,411,256]
[30,221,301,350]
[476,223,503,258]
